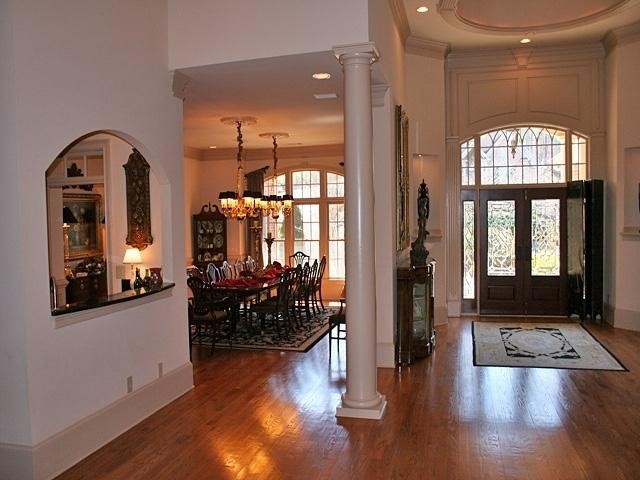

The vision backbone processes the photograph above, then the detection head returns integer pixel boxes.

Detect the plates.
[213,235,223,248]
[214,221,224,233]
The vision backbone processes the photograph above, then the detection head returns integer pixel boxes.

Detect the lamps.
[219,116,295,224]
[122,247,143,285]
[511,139,516,159]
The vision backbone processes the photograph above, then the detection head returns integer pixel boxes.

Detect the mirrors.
[44,129,176,317]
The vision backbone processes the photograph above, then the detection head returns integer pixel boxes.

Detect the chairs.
[329,281,347,364]
[187,250,327,365]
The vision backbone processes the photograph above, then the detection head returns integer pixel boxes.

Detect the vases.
[133,266,143,290]
[150,267,164,292]
[142,268,151,291]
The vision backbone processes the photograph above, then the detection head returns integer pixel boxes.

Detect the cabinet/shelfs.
[396,255,438,371]
[191,200,228,272]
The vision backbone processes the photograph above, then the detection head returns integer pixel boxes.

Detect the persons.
[415,185,430,248]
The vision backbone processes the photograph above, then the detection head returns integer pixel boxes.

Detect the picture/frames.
[394,103,411,252]
[62,191,105,261]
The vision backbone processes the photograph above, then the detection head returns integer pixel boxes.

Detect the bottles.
[134,267,152,288]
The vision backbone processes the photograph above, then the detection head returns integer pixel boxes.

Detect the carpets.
[470,321,630,372]
[190,303,346,353]
